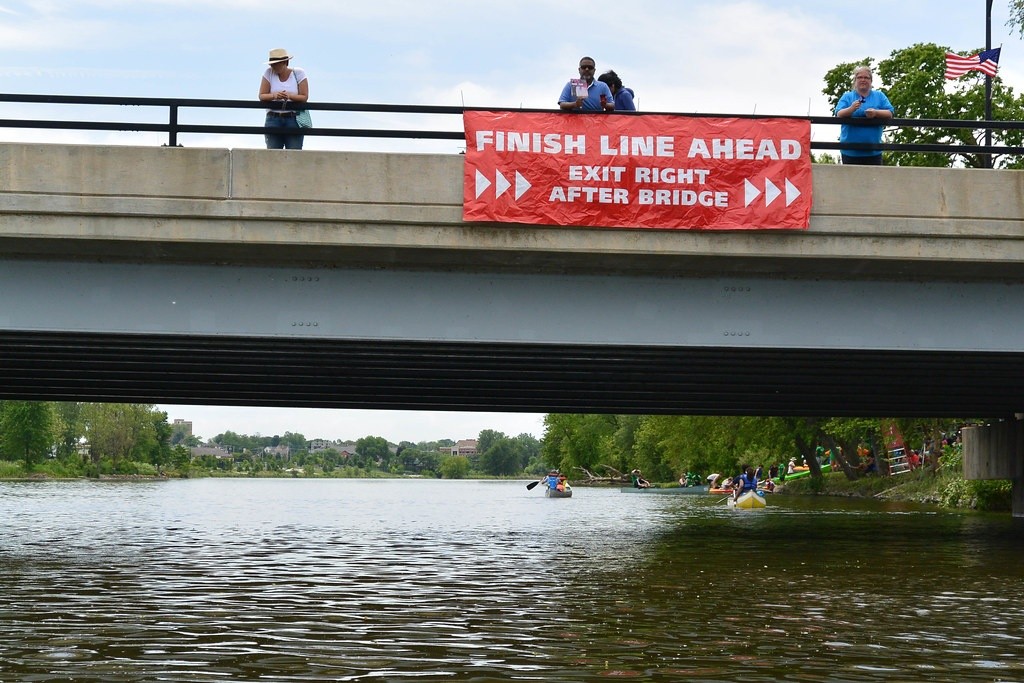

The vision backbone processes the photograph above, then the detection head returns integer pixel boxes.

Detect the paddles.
[638,485,656,489]
[716,494,733,504]
[527,479,542,490]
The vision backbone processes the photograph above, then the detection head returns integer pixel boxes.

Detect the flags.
[944,47,1001,77]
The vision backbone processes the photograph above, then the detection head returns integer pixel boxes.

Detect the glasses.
[579,64,595,70]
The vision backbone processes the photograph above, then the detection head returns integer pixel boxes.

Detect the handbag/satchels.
[294,107,313,129]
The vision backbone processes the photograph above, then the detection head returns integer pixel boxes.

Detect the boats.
[771,464,832,485]
[621,484,710,495]
[545,483,572,498]
[792,446,869,473]
[709,487,772,494]
[727,489,766,508]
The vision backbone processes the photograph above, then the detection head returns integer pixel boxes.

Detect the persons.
[542,470,567,492]
[259,48,309,150]
[679,426,962,500]
[598,70,636,111]
[835,66,894,166]
[636,474,650,489]
[632,469,641,488]
[558,56,615,110]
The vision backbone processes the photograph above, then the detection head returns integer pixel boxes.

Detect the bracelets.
[273,93,274,100]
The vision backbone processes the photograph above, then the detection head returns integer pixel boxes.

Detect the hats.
[268,48,292,65]
[790,457,796,460]
[550,470,557,476]
[559,473,564,475]
